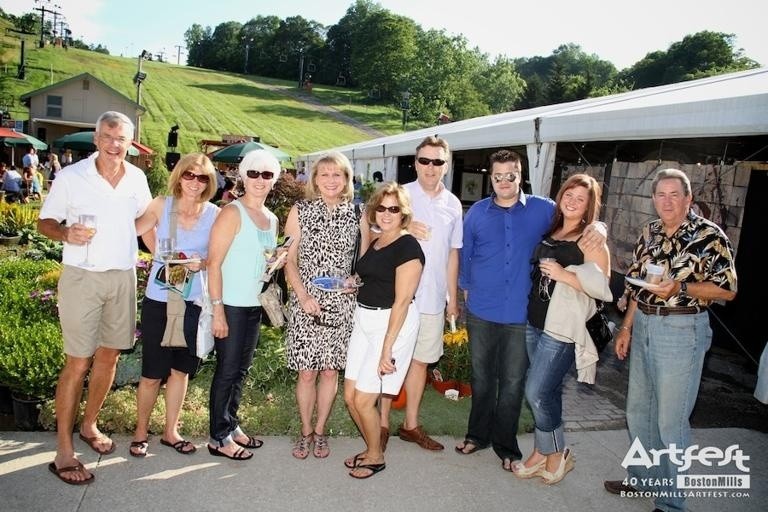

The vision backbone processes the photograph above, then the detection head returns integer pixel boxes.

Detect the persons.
[36,111,158,486]
[0,146,73,205]
[372,134,463,450]
[206,150,292,462]
[282,150,371,461]
[510,173,614,486]
[131,151,223,459]
[343,183,427,480]
[209,156,310,209]
[605,165,740,511]
[459,149,610,471]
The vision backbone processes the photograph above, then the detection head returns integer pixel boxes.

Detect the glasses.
[417,158,446,166]
[491,172,517,183]
[538,276,553,301]
[246,170,274,180]
[181,170,210,183]
[375,204,400,213]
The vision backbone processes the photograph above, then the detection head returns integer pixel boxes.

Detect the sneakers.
[604,479,642,498]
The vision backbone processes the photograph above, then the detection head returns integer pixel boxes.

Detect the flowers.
[434,326,474,383]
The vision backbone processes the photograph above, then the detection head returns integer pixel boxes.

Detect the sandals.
[207,443,253,460]
[513,448,547,478]
[455,438,489,453]
[291,431,313,460]
[502,455,516,472]
[542,446,573,484]
[234,437,264,448]
[349,463,386,480]
[345,454,365,467]
[312,429,330,458]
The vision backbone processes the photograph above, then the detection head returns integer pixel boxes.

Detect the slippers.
[160,435,196,455]
[79,433,116,454]
[129,439,149,457]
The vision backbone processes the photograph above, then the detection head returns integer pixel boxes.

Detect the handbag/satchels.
[259,270,285,328]
[196,294,215,358]
[585,311,613,356]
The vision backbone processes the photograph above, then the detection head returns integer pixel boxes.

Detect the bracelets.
[211,298,223,308]
[618,324,632,332]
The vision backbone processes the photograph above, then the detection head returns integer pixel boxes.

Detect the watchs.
[678,280,688,297]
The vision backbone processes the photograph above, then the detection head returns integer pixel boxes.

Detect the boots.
[49,461,94,485]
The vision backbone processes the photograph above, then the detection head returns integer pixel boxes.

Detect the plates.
[153,249,203,263]
[311,276,350,292]
[623,275,655,288]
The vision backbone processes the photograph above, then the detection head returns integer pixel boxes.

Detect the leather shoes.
[378,425,390,451]
[399,422,445,452]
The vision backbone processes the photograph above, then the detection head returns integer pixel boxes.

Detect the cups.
[648,264,667,284]
[539,257,556,275]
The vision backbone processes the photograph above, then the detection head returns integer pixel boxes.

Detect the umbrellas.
[53,125,140,163]
[0,127,48,167]
[207,140,293,165]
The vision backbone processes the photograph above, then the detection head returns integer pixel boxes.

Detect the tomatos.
[180,251,187,259]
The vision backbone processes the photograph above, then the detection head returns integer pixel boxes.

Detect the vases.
[426,368,472,397]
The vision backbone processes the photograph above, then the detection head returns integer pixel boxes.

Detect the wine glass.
[157,237,176,289]
[76,214,98,268]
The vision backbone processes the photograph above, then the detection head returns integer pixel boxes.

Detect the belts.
[636,300,706,315]
